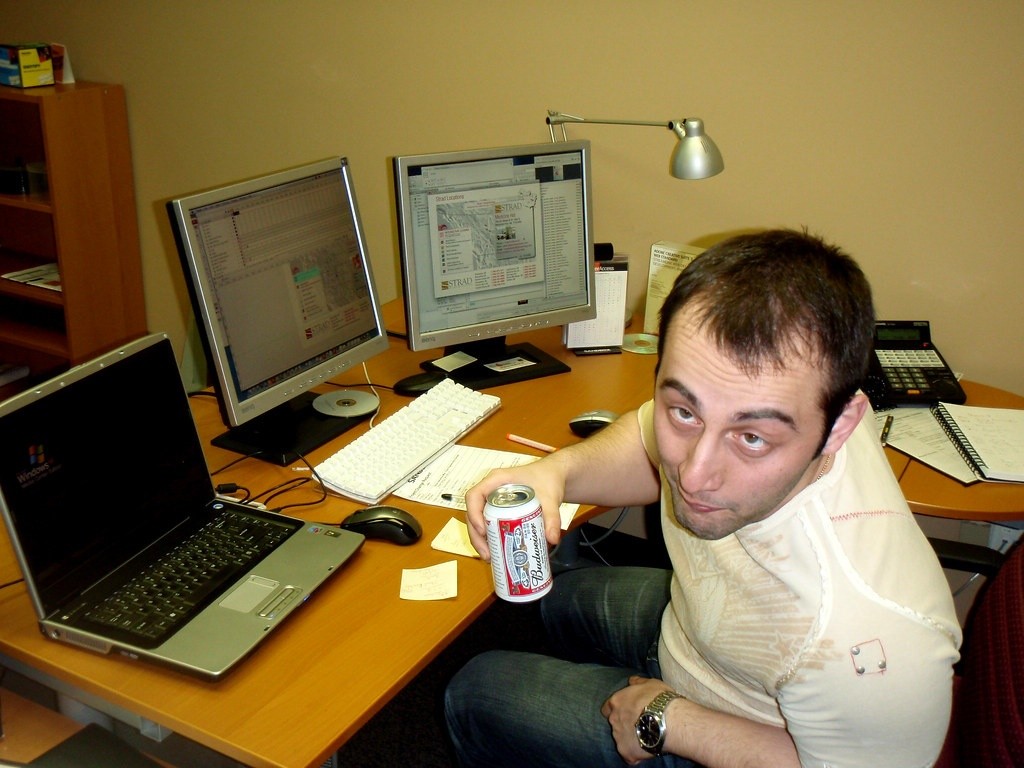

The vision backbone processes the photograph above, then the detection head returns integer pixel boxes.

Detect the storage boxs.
[0,82,148,367]
[0,42,54,87]
[0,362,33,400]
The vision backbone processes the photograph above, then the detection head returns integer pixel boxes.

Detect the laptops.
[0,334,367,678]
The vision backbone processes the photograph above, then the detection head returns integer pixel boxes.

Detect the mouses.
[567,409,617,438]
[340,505,423,546]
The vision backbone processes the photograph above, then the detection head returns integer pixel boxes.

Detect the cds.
[313,389,380,417]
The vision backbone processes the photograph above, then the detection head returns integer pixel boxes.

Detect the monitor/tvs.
[394,141,598,389]
[165,155,391,466]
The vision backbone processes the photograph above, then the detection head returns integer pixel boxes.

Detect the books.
[927,401,1024,484]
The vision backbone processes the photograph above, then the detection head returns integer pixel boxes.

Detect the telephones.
[861,320,967,410]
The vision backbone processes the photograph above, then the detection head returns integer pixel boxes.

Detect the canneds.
[484,483,553,603]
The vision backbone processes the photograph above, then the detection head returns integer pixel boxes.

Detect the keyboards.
[311,379,503,505]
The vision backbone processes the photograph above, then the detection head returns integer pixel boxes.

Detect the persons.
[442,231,962,768]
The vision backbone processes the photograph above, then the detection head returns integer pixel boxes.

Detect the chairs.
[930,537,1024,768]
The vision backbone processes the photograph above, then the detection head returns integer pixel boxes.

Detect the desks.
[0,303,1024,768]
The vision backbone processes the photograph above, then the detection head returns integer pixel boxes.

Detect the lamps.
[547,114,723,183]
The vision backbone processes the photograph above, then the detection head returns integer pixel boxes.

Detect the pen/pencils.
[880,415,893,446]
[506,433,558,453]
[441,494,465,502]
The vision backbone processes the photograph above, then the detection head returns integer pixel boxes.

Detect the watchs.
[633,690,686,758]
